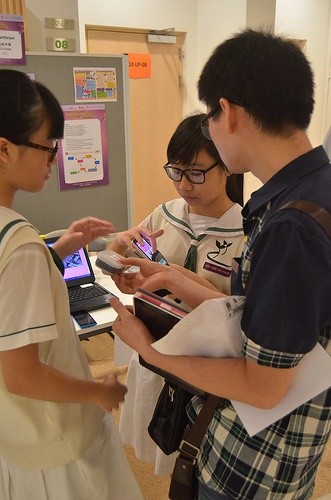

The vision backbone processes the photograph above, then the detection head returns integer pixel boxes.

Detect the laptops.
[42,236,118,317]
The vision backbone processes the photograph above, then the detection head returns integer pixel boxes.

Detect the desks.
[71,231,135,341]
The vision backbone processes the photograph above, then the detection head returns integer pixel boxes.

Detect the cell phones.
[130,236,168,266]
[72,309,97,329]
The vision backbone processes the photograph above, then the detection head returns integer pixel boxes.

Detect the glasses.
[7,138,60,163]
[163,160,222,184]
[200,98,244,140]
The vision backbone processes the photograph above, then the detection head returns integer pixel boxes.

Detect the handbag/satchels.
[168,456,201,500]
[147,379,193,456]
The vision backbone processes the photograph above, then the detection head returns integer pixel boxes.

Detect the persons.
[106,113,247,477]
[107,31,331,500]
[0,67,145,500]
